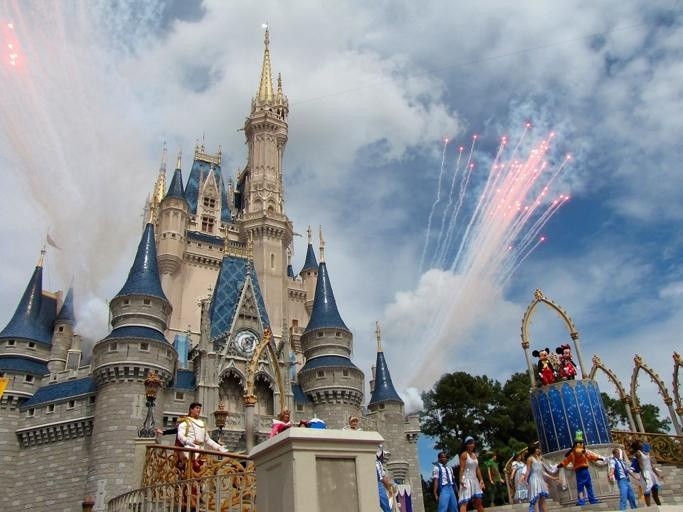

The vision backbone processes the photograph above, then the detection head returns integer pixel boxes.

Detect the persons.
[482,443,561,512]
[460,435,486,512]
[430,451,459,512]
[156,402,401,512]
[608,439,665,512]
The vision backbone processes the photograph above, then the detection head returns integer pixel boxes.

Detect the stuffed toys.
[555,343,581,381]
[556,430,605,506]
[532,346,558,385]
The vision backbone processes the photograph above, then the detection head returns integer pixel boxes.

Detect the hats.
[464,435,474,444]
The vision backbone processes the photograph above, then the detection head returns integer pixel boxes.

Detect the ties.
[619,460,631,481]
[444,467,451,486]
